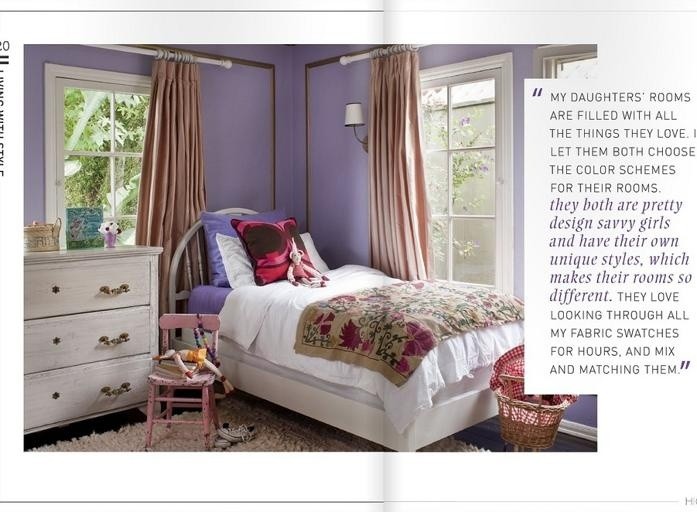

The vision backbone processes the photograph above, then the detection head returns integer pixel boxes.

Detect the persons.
[151,347,234,394]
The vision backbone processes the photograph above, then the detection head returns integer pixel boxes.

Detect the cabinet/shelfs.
[25,245,163,435]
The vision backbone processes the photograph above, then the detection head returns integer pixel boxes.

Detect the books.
[152,360,202,384]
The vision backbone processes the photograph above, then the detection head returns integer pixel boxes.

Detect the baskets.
[24,218,61,252]
[494,374,572,450]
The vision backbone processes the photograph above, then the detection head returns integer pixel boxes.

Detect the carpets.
[26,395,493,451]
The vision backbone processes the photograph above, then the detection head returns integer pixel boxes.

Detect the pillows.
[198,212,330,290]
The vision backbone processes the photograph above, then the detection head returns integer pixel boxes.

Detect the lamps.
[345,102,367,155]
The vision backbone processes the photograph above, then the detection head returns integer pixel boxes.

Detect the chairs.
[145,313,220,451]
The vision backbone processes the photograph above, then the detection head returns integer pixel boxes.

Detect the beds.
[168,208,527,452]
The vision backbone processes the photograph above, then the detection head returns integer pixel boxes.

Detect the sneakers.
[217,422,258,443]
[214,423,231,449]
